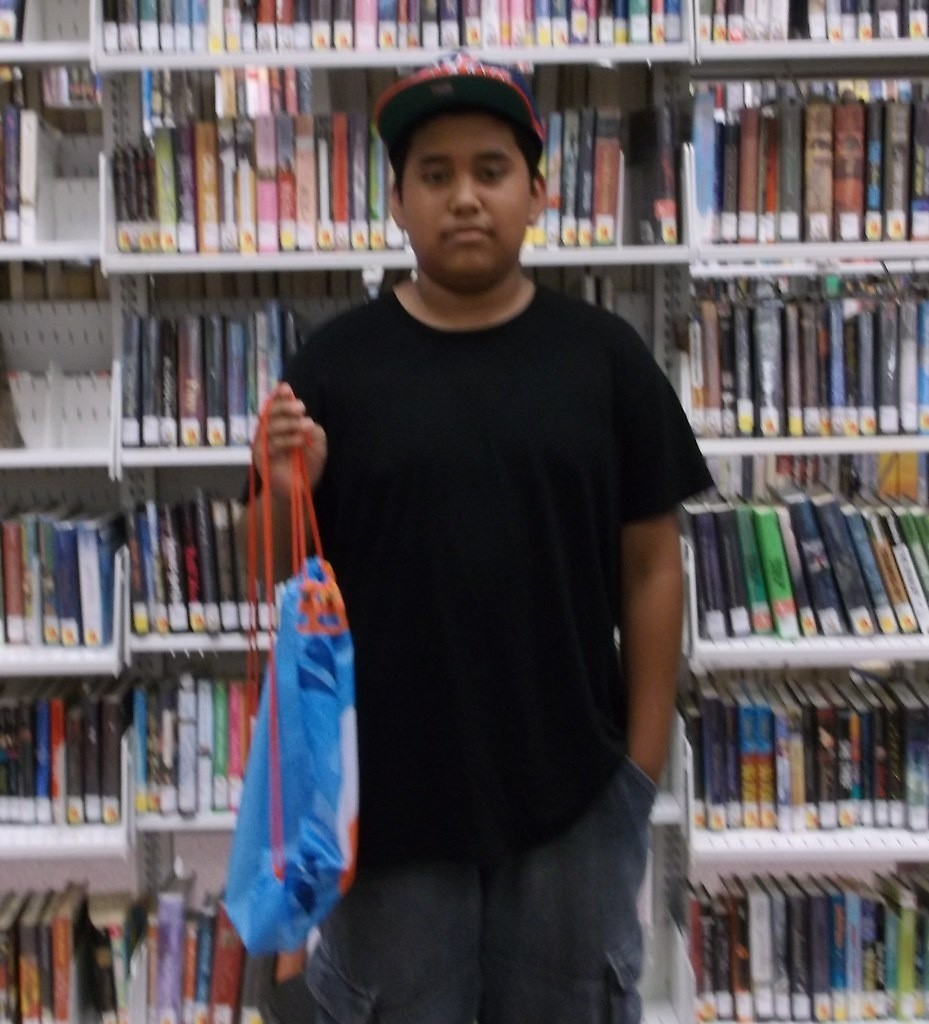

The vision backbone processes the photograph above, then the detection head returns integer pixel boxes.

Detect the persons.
[237,54,719,1023]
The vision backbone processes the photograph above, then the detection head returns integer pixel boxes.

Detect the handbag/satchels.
[225,392,360,956]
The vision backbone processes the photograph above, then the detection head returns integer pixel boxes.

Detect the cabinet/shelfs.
[0,0,929,1024]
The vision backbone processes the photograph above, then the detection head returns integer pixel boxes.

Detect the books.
[1,2,929,1024]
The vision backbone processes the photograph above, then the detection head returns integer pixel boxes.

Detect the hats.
[374,50,548,168]
